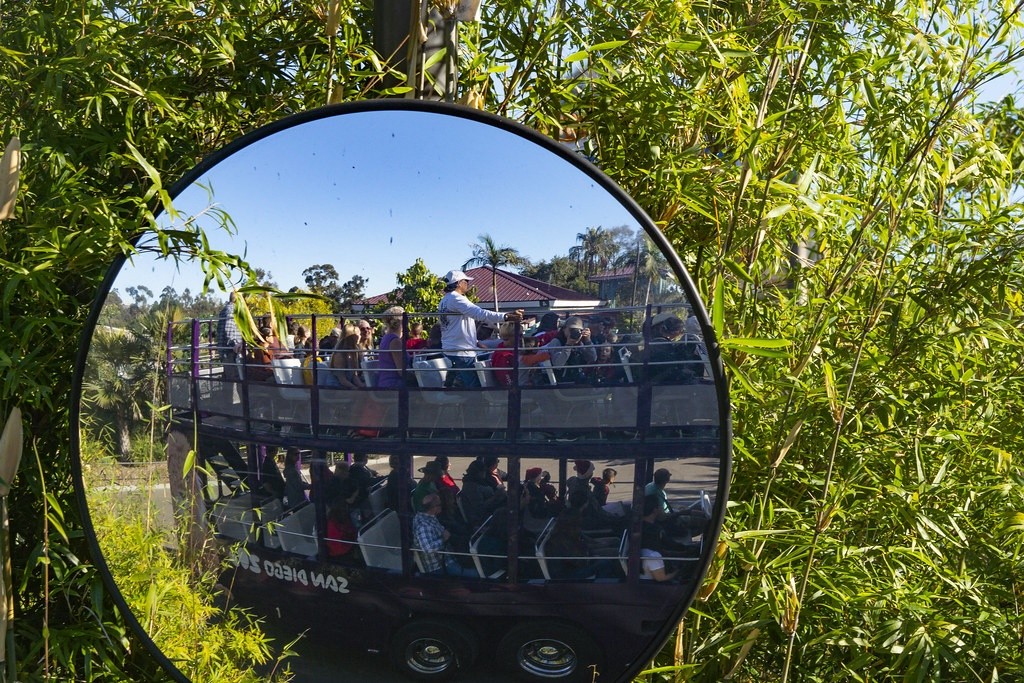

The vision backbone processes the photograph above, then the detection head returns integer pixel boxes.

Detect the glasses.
[270,335,276,338]
[360,326,372,331]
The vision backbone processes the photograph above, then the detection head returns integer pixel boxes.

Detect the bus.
[164,306,724,682]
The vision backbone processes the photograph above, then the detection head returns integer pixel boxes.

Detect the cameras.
[570,328,583,339]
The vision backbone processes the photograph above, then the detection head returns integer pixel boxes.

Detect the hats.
[419,493,438,510]
[444,270,475,285]
[418,460,447,476]
[650,311,673,326]
[565,316,583,330]
[685,315,702,334]
[654,468,673,483]
[523,467,542,481]
[572,459,594,480]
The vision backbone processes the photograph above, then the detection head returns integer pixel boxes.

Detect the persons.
[637,468,710,582]
[437,270,529,387]
[262,444,288,504]
[283,446,314,516]
[248,305,443,389]
[307,449,385,567]
[410,456,480,577]
[388,454,416,514]
[459,454,633,578]
[214,291,246,379]
[489,310,707,387]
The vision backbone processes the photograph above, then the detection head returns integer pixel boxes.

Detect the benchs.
[236,342,714,383]
[209,477,712,580]
